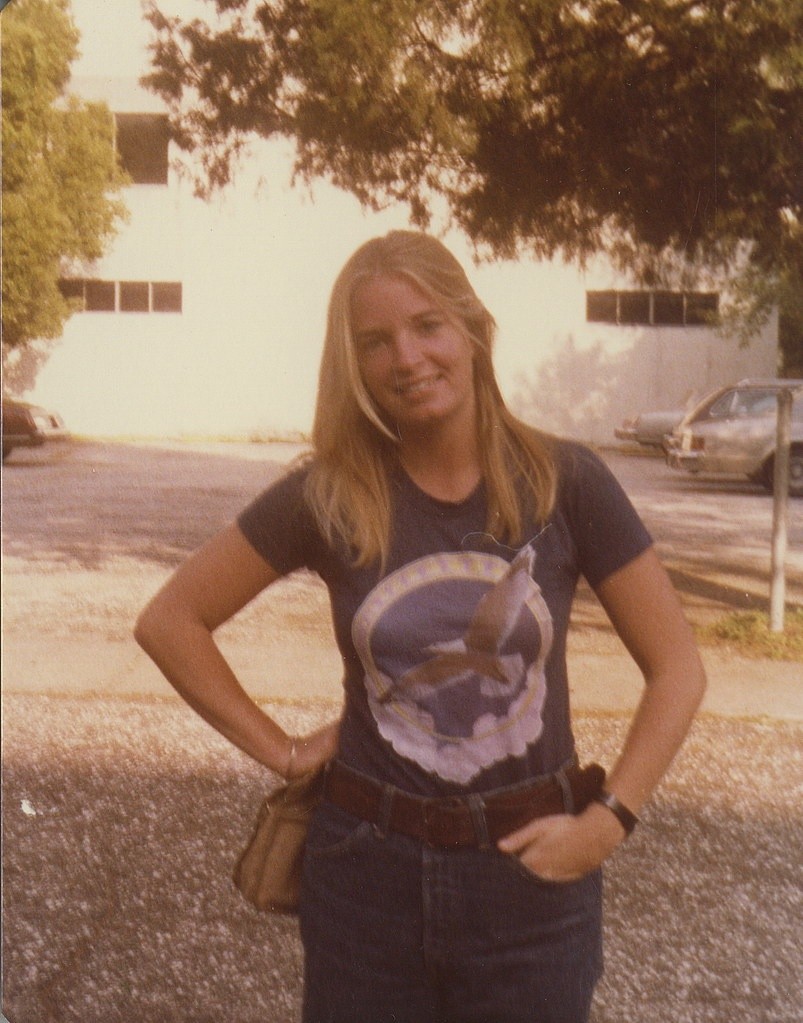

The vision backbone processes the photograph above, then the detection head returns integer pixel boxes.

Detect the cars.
[615,375,803,497]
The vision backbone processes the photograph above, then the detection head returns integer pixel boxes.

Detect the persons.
[134,231,706,1023]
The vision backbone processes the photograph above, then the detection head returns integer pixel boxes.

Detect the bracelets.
[595,789,639,833]
[285,737,297,777]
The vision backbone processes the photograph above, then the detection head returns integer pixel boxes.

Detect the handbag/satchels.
[232,778,315,914]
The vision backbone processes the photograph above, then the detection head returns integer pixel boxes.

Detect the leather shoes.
[324,762,607,847]
